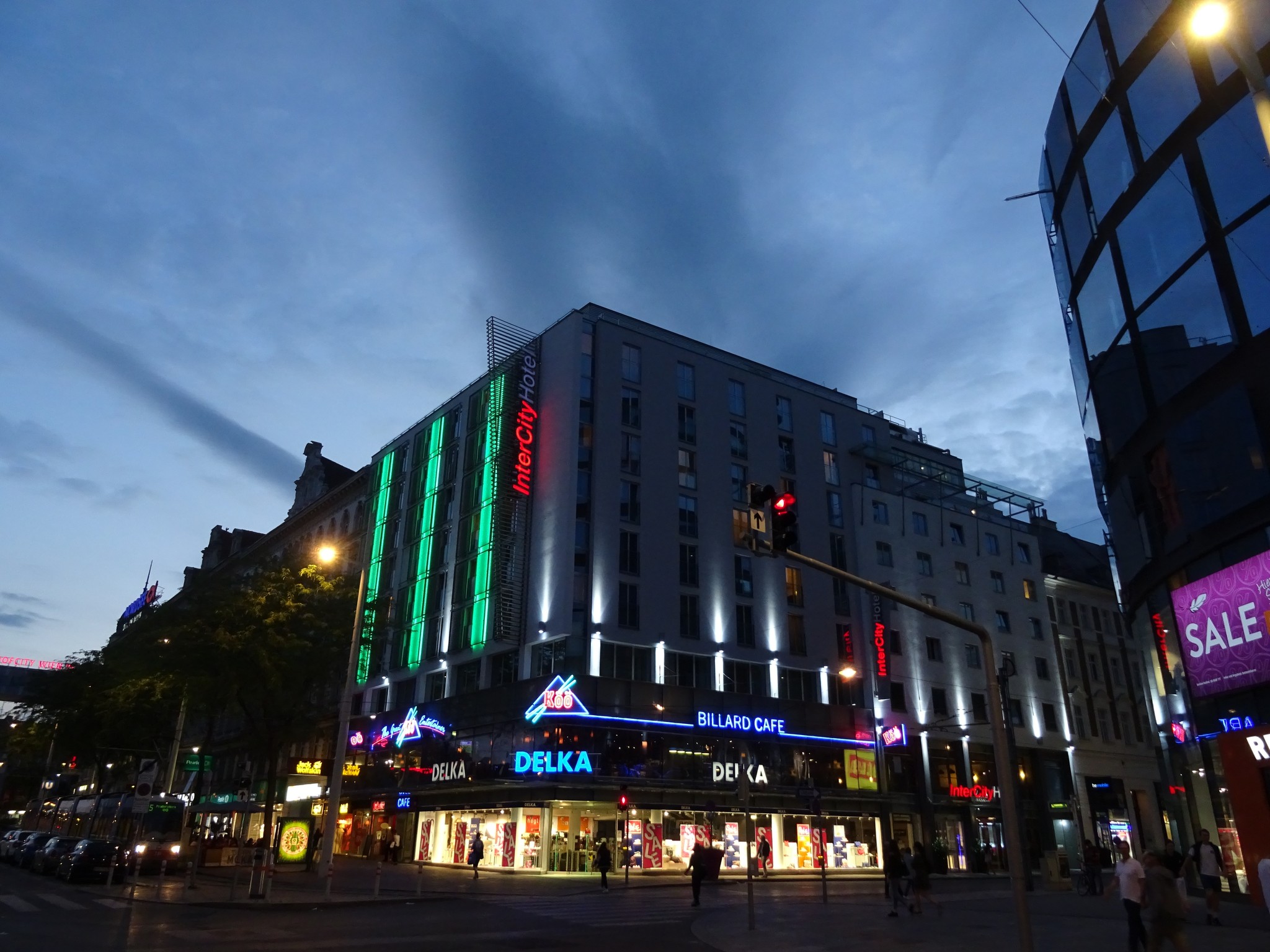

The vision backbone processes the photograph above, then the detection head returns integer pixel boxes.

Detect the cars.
[0,829,127,885]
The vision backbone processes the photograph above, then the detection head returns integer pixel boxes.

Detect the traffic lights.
[66,755,77,770]
[747,481,800,549]
[619,795,628,812]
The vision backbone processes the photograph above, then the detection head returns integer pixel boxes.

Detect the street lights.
[311,542,369,884]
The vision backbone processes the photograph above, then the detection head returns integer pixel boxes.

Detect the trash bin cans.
[249,848,274,900]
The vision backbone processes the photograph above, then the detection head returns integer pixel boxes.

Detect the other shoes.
[1213,917,1221,925]
[1205,914,1212,925]
[603,890,608,893]
[888,911,898,916]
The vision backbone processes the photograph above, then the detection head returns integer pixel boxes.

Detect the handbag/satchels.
[468,849,476,864]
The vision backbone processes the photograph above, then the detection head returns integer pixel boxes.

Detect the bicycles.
[1075,858,1103,896]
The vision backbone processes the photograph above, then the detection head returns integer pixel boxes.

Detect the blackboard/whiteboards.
[362,835,373,856]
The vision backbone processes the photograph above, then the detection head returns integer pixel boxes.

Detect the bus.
[23,790,185,876]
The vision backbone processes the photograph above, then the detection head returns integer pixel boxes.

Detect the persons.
[471,833,484,878]
[389,829,400,866]
[685,843,707,906]
[883,839,929,917]
[1084,839,1104,895]
[1102,829,1230,952]
[984,842,996,875]
[594,842,612,893]
[757,835,770,877]
